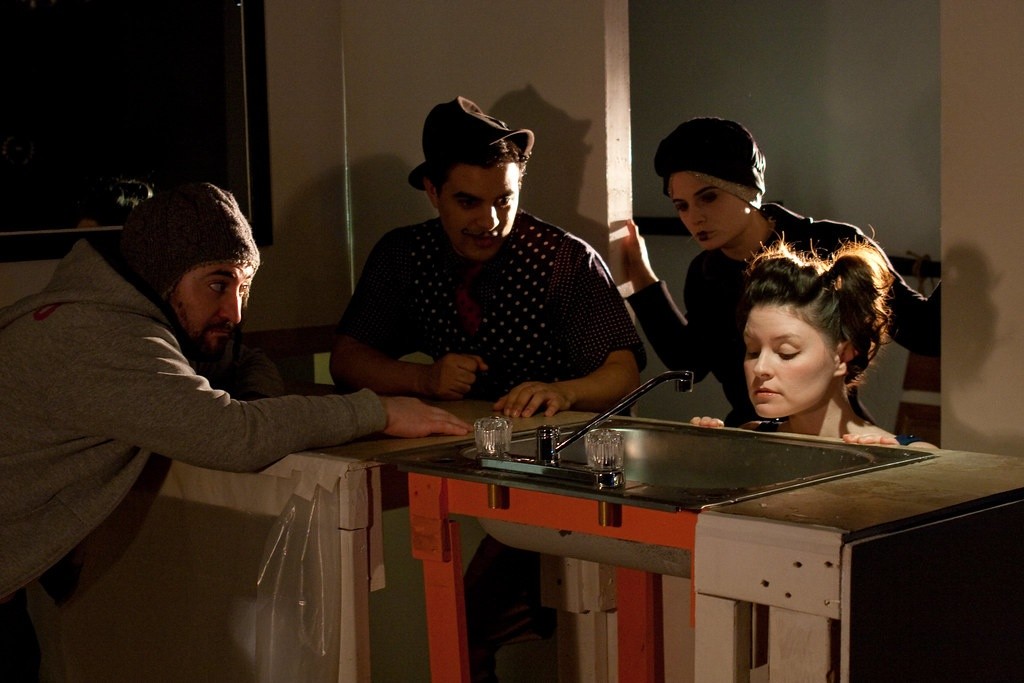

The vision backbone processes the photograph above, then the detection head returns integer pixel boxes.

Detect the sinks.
[371,415,946,582]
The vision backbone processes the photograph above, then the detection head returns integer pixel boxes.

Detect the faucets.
[472,369,695,489]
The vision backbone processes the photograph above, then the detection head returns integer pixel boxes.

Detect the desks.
[23,384,599,683]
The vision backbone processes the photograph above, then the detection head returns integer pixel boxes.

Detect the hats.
[408,96,537,191]
[122,184,261,301]
[654,118,766,210]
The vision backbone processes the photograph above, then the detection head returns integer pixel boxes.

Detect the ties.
[455,263,482,336]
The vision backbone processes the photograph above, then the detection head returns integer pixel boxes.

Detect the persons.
[0,181,473,683]
[689,239,939,449]
[623,117,943,431]
[328,97,648,683]
